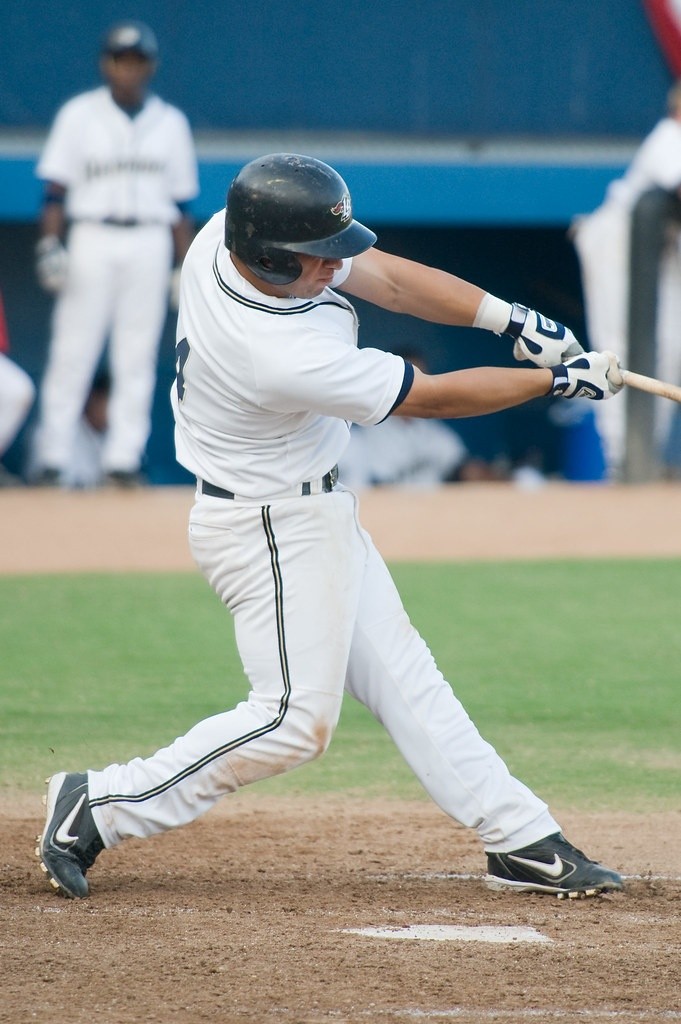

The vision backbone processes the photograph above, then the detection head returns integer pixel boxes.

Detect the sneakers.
[31,769,104,900]
[481,831,627,899]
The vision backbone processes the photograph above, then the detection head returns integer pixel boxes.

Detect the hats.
[101,22,161,60]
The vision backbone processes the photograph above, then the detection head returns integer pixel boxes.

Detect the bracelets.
[44,192,65,208]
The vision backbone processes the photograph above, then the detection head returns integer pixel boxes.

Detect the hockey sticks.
[564,355,681,405]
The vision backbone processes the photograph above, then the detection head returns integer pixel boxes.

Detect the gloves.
[551,350,627,402]
[169,265,185,310]
[34,234,72,292]
[501,302,586,368]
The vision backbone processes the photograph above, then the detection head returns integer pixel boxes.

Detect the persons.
[32,153,625,900]
[0,24,681,491]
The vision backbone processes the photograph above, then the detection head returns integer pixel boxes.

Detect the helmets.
[223,151,378,286]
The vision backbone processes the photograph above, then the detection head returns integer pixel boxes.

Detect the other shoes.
[33,464,74,488]
[111,469,149,489]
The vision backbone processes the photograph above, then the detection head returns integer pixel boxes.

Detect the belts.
[195,465,343,500]
